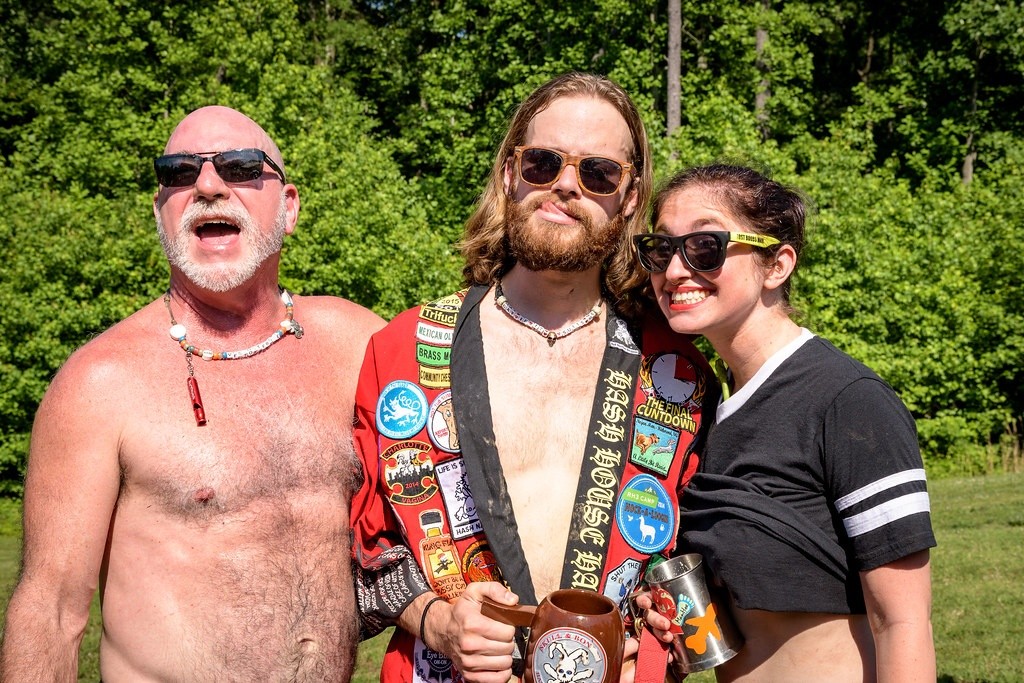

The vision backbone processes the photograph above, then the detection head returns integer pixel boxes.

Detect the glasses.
[153,148,286,188]
[513,145,639,197]
[631,230,781,274]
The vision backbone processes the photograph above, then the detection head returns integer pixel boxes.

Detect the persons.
[0,104,394,683]
[630,164,942,682]
[343,73,721,683]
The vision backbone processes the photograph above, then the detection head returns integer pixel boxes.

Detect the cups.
[476,587,623,683]
[628,552,746,673]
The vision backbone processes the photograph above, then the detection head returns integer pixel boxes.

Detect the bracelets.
[420,597,448,647]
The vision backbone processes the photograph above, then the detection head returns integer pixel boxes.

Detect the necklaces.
[495,278,603,344]
[162,285,303,427]
